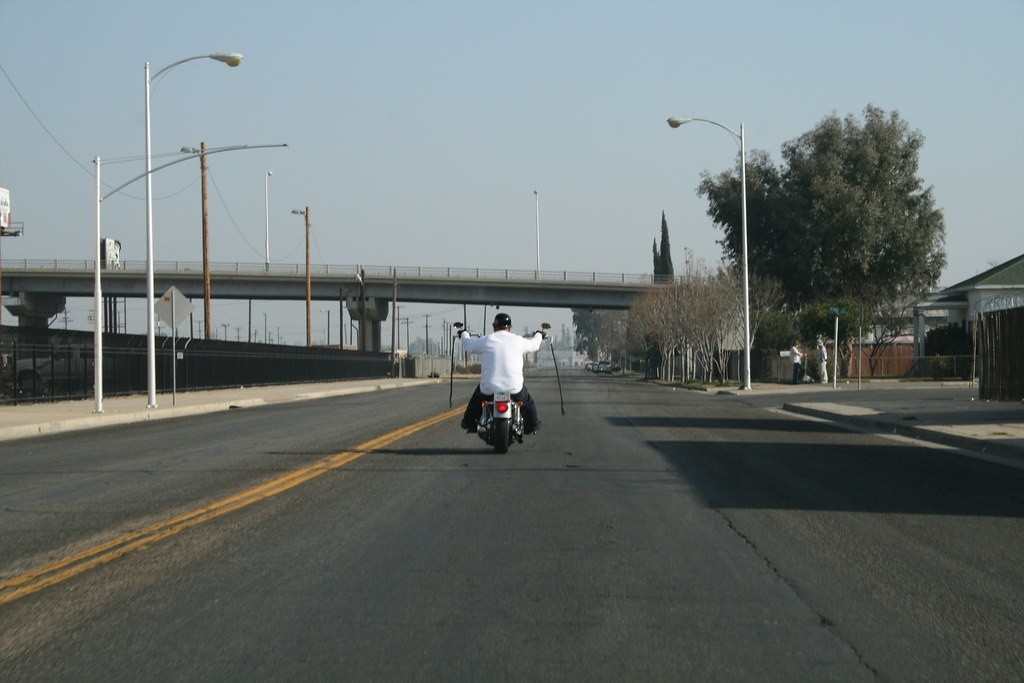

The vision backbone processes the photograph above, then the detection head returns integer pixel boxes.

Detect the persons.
[789,340,807,385]
[813,339,829,384]
[457,313,548,434]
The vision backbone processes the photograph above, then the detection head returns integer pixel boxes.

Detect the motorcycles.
[453,323,554,451]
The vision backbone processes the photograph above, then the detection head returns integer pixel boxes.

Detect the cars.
[586,362,598,371]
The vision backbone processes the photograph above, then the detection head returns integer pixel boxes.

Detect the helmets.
[492,314,512,328]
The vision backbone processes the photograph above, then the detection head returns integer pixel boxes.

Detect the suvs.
[598,361,612,373]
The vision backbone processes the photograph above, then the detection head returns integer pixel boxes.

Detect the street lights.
[265,170,274,275]
[319,309,330,344]
[146,52,245,408]
[534,189,540,280]
[180,146,210,342]
[668,116,752,390]
[292,208,311,349]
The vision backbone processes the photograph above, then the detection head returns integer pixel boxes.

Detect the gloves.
[533,331,546,339]
[457,330,466,339]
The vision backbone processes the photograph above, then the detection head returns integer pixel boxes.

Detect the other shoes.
[525,420,542,434]
[461,418,476,430]
[821,380,828,384]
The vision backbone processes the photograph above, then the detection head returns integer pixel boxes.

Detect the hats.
[816,341,823,346]
